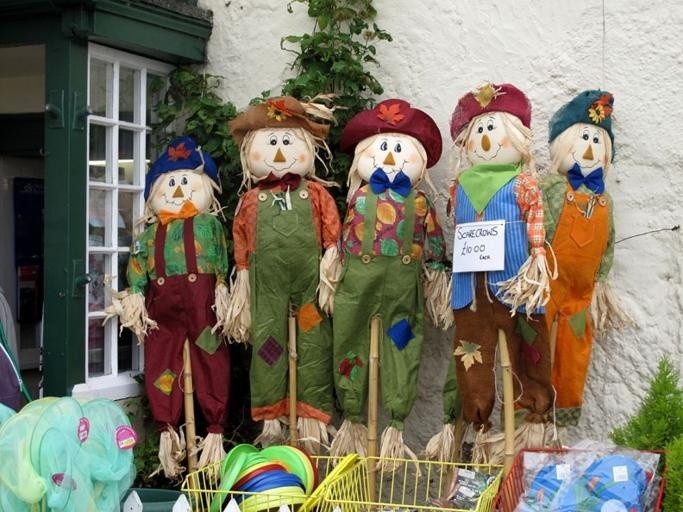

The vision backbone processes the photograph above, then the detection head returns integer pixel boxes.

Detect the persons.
[100,86,616,482]
[99,85,618,480]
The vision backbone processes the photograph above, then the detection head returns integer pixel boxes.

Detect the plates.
[209,443,319,511]
